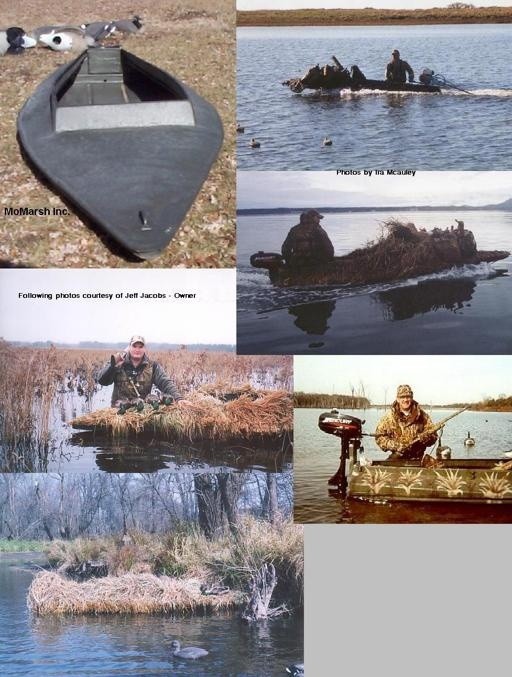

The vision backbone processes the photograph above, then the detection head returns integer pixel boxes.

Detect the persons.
[385,50,414,82]
[281,208,334,267]
[374,384,437,460]
[96,335,195,408]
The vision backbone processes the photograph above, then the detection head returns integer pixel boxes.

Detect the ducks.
[164,640,209,659]
[285,663,305,677]
[202,584,230,596]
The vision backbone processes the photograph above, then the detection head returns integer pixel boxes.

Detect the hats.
[308,210,324,219]
[130,336,144,345]
[397,385,412,397]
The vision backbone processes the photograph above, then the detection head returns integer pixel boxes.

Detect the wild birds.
[436,437,451,456]
[236,124,245,132]
[249,138,260,147]
[464,431,475,446]
[324,137,333,146]
[116,393,173,415]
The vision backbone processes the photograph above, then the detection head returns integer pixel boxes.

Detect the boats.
[317,409,511,503]
[280,64,442,92]
[16,46,222,259]
[72,386,295,440]
[248,223,511,286]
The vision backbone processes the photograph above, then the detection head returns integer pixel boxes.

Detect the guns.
[387,406,471,460]
